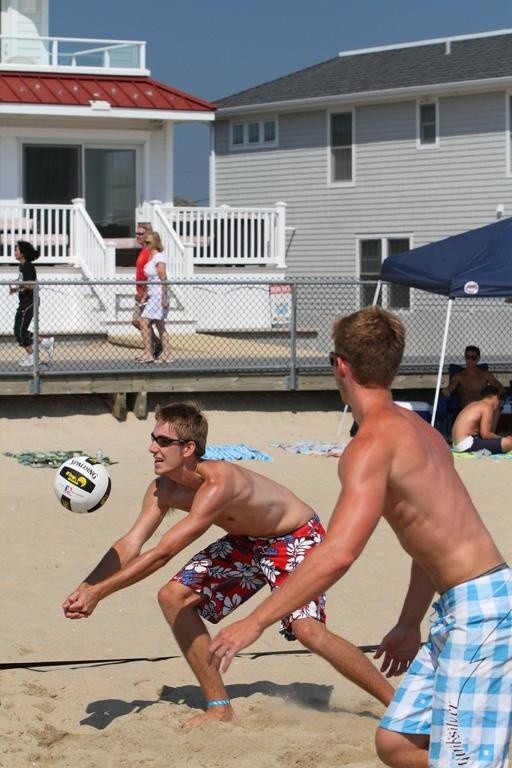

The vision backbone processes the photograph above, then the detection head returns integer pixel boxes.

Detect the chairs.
[448,363,488,435]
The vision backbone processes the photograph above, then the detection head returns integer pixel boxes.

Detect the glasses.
[466,354,478,360]
[330,351,346,365]
[151,432,199,447]
[136,232,150,244]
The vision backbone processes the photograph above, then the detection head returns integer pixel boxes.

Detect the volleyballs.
[55,457,111,512]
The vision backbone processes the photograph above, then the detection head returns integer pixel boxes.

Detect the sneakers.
[40,337,54,358]
[19,352,41,366]
[134,352,176,363]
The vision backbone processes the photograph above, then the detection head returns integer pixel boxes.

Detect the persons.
[206,306,512,766]
[440,346,504,414]
[136,232,175,363]
[61,398,398,732]
[451,386,512,457]
[10,239,55,369]
[130,220,165,363]
